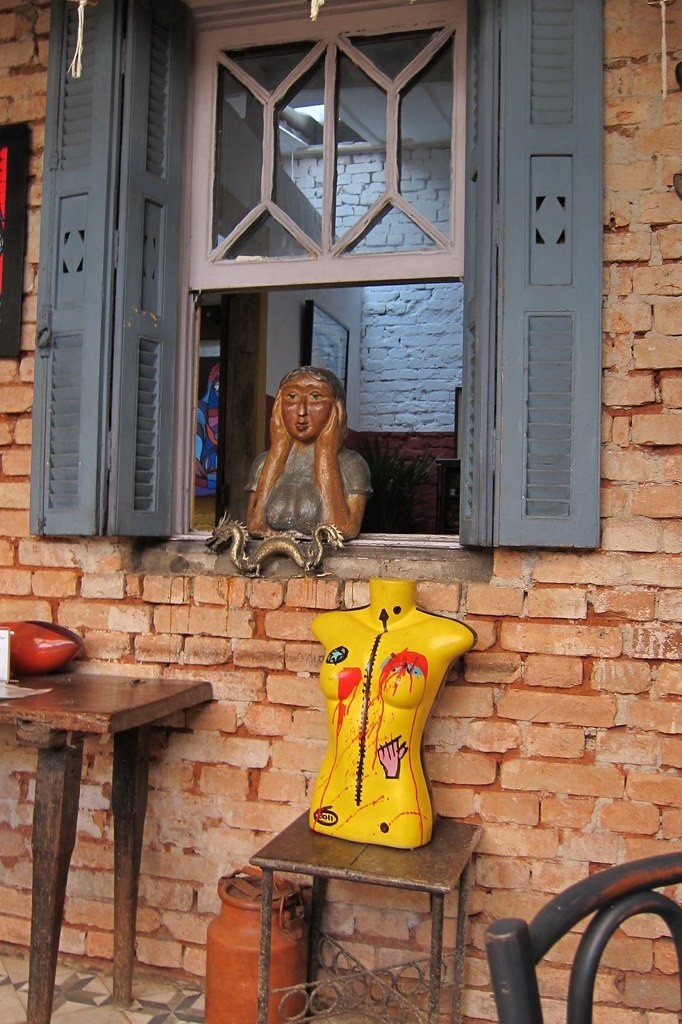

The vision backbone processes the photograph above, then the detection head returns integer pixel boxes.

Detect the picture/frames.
[0,122,30,358]
[304,300,350,394]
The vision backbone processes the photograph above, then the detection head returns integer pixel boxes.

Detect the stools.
[249,808,483,1024]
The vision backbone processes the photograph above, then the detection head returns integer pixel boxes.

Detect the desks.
[0,671,213,1024]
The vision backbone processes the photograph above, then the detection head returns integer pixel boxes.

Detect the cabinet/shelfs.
[436,458,460,533]
